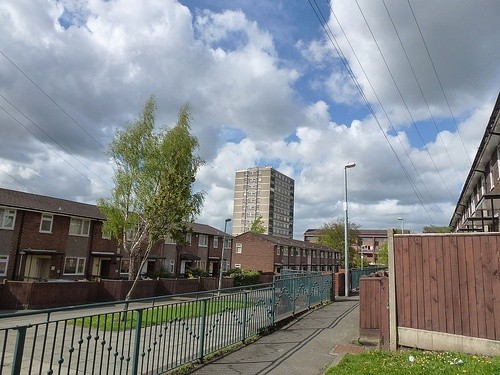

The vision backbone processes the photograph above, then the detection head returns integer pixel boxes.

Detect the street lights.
[397,218,404,235]
[218,218,231,298]
[344,164,357,296]
